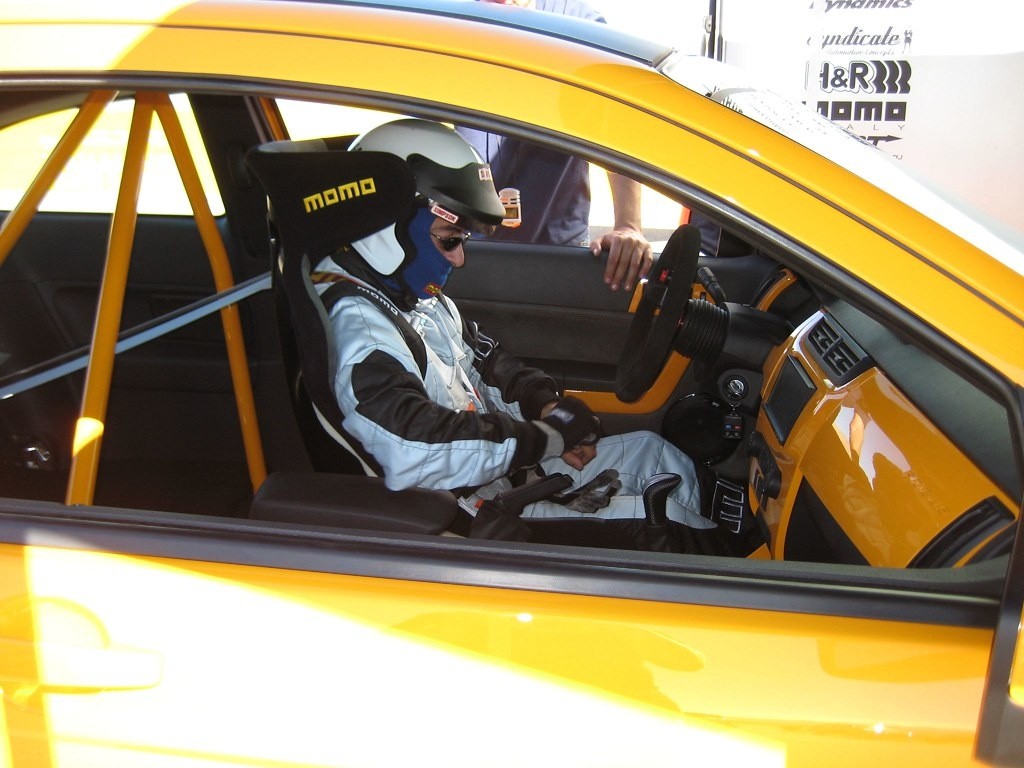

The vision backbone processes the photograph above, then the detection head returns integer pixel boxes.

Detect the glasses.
[430,232,470,252]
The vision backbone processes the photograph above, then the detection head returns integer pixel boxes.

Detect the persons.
[455,120,656,292]
[308,115,763,558]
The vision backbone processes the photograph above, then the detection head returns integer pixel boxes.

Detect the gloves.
[543,395,600,453]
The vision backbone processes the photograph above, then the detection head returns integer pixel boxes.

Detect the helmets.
[335,119,506,276]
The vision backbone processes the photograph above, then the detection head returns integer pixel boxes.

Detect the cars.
[0,0,1024,768]
